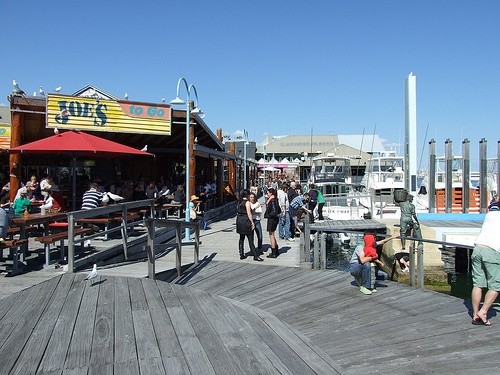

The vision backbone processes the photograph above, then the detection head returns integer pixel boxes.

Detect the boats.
[304,153,498,228]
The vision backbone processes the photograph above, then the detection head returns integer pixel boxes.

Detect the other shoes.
[359,285,372,294]
[257,248,266,254]
[281,237,295,241]
[253,257,263,261]
[371,288,377,292]
[239,255,248,259]
[87,223,100,232]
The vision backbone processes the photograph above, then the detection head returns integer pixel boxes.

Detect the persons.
[350,232,400,294]
[250,191,264,255]
[471,195,500,325]
[264,187,282,257]
[0,176,61,255]
[393,194,423,250]
[250,172,325,241]
[236,190,264,262]
[59,168,207,226]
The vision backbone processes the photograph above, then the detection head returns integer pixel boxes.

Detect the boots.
[266,245,279,258]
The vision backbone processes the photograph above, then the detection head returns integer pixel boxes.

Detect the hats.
[20,187,27,193]
[190,194,199,201]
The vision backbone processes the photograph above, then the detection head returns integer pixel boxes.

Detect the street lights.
[170,77,204,242]
[240,129,249,190]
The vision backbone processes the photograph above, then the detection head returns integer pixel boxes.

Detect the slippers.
[472,311,491,325]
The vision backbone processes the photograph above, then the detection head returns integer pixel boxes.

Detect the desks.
[10,212,67,268]
[163,204,183,218]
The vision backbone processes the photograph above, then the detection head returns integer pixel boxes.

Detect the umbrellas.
[8,130,155,211]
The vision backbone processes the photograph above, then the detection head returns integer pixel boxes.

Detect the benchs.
[0,211,144,276]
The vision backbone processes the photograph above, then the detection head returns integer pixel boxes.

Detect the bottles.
[24,207,28,219]
[41,203,45,215]
